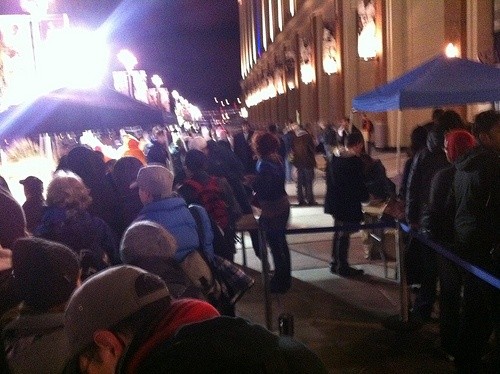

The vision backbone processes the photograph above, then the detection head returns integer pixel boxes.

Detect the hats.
[448,130,477,161]
[121,221,176,264]
[20,176,43,191]
[189,136,207,150]
[129,164,174,195]
[12,238,81,306]
[58,264,170,374]
[183,150,205,167]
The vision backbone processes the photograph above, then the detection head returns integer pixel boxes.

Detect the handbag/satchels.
[178,249,220,298]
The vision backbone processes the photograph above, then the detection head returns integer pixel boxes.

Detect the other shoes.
[331,265,363,275]
[435,337,457,363]
[300,199,318,205]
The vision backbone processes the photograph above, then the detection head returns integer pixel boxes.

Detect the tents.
[353,56,499,191]
[0,88,177,148]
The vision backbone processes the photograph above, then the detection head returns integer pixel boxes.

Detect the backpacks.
[187,178,229,229]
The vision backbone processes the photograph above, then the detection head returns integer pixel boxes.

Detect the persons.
[65,265,326,374]
[0,110,475,360]
[452,113,500,374]
[0,238,80,374]
